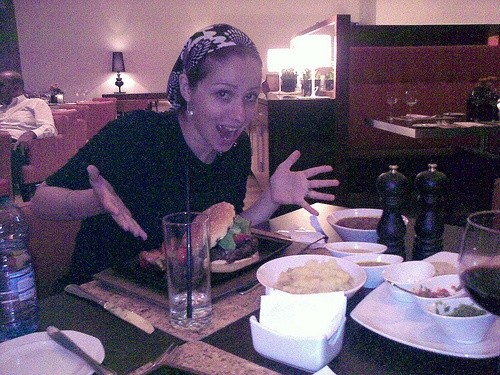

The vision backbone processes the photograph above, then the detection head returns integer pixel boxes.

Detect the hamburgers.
[178,202,259,274]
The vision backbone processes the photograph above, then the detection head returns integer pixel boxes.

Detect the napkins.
[260,289,346,339]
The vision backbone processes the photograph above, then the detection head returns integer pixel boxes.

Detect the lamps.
[112,52,126,95]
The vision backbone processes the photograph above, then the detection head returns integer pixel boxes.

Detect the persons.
[0,70,58,173]
[26,23,339,286]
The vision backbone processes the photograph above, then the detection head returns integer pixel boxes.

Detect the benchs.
[348,45,500,149]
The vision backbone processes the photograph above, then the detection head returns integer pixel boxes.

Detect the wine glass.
[75,88,88,102]
[386,86,417,122]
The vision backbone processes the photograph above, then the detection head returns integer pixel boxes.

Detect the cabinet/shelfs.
[248,93,333,190]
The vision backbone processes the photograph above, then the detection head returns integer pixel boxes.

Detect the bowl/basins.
[256,208,495,344]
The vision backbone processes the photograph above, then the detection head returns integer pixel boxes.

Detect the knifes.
[64,285,155,334]
[47,325,117,375]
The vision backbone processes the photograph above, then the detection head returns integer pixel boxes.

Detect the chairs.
[0,98,117,197]
[322,144,500,230]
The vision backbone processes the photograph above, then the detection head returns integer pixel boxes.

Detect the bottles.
[1,178,39,339]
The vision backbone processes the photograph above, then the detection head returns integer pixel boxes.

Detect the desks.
[365,117,500,154]
[37,202,500,375]
[102,93,167,112]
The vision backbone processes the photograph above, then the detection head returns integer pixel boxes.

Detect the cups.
[164,212,212,330]
[457,211,500,316]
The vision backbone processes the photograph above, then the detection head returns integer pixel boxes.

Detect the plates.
[1,332,104,375]
[351,251,500,358]
[209,231,293,273]
